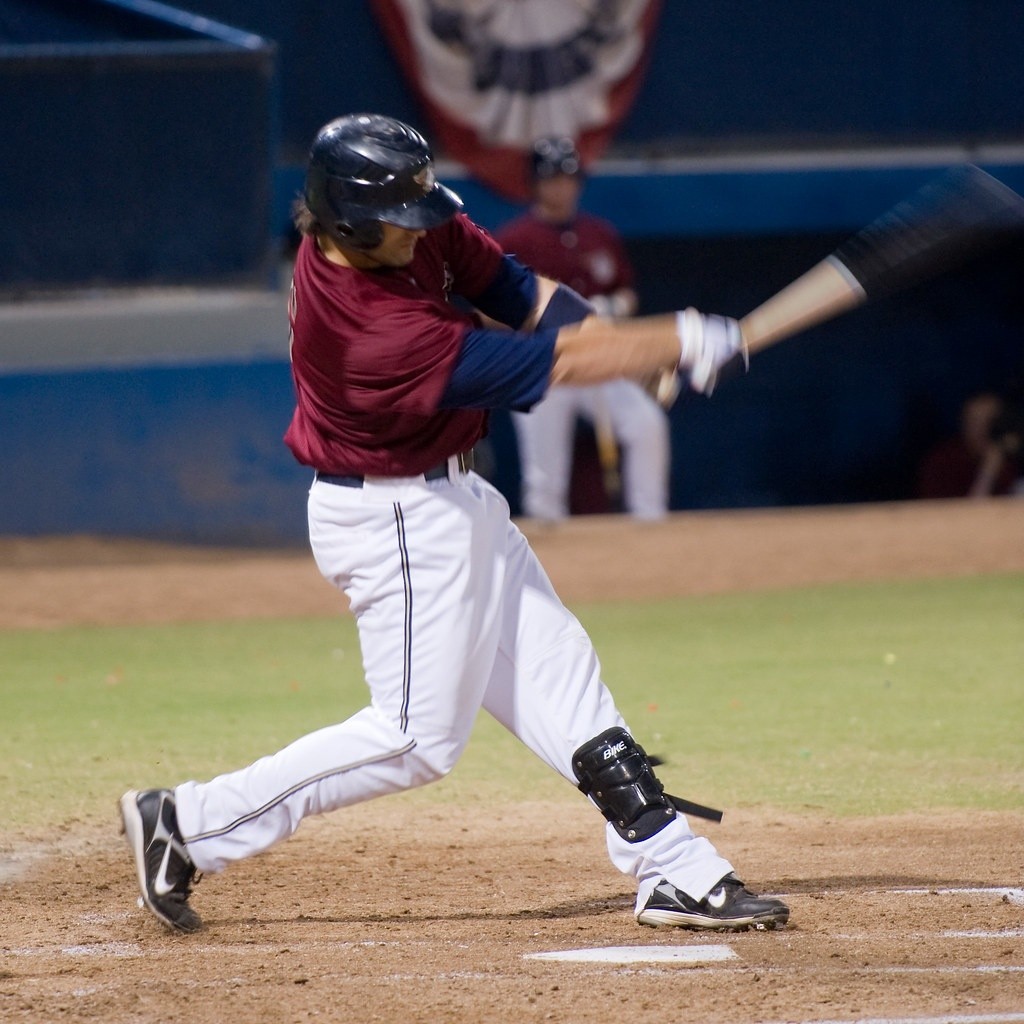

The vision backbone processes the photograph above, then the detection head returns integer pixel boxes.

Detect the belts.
[316,447,475,487]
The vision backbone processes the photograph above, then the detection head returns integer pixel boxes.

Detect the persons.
[471,137,672,521]
[115,112,791,935]
[963,349,1024,500]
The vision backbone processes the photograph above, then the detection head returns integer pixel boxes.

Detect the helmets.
[522,134,584,185]
[304,114,465,250]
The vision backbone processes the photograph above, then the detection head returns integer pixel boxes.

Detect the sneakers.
[636,869,791,929]
[119,787,204,933]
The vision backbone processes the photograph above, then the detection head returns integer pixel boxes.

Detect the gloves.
[674,307,743,396]
[585,291,635,320]
[633,365,681,410]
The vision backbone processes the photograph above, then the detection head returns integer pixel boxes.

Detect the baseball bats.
[649,165,1022,406]
